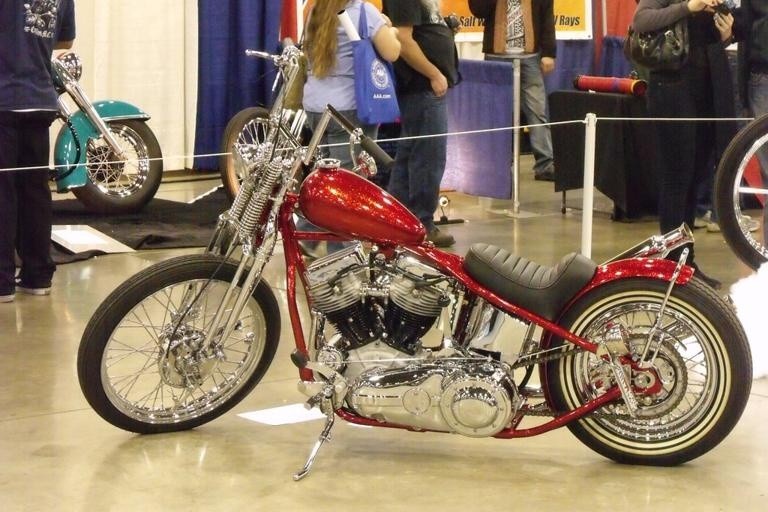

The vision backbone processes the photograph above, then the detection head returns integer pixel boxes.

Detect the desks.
[547,88,659,224]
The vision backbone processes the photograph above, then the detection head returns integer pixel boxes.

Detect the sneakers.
[425,228,455,247]
[689,264,723,293]
[0,275,54,304]
[693,210,760,232]
[535,162,556,182]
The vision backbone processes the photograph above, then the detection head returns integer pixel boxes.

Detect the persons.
[293,0,401,258]
[382,0,458,248]
[467,0,556,181]
[0,0,75,303]
[633,0,768,289]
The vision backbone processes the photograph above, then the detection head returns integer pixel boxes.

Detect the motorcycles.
[220,44,306,210]
[77,37,754,486]
[49,50,165,217]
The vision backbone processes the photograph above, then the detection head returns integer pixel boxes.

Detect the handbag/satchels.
[628,22,691,74]
[351,2,402,125]
[393,23,462,93]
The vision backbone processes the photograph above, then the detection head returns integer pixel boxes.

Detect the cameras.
[712,0,735,21]
